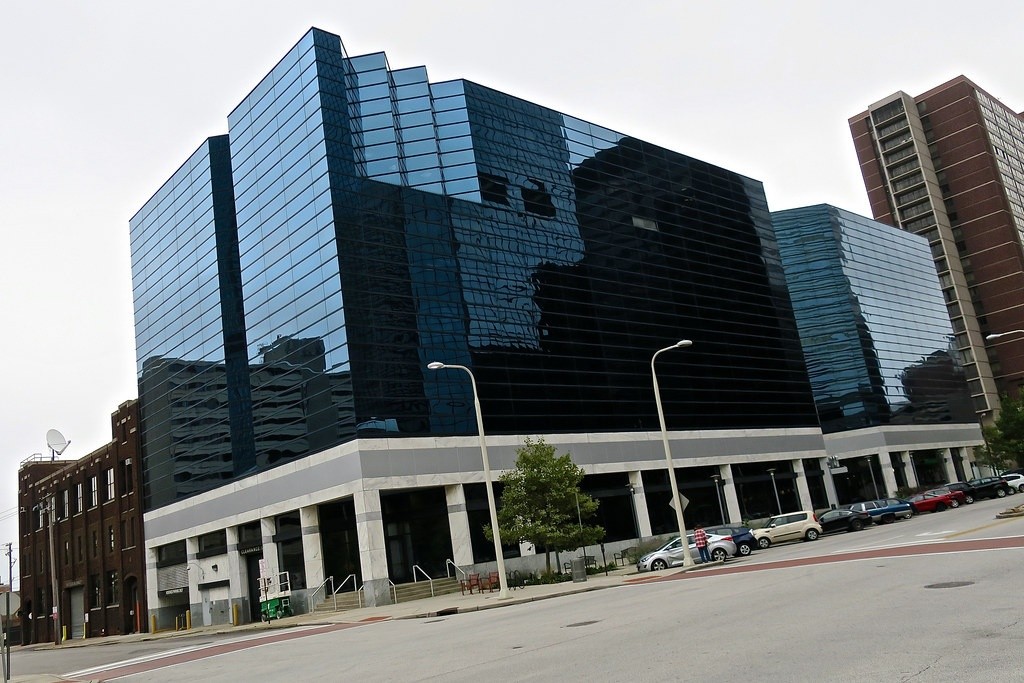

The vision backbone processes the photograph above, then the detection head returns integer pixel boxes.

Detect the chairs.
[479,572,500,594]
[460,573,480,596]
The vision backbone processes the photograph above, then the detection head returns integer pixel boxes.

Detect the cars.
[636,533,737,573]
[905,494,951,513]
[818,509,873,533]
[937,481,982,504]
[873,498,913,519]
[919,488,964,509]
[967,476,1010,498]
[1002,473,1024,493]
[705,528,760,557]
[840,501,896,525]
[750,510,822,549]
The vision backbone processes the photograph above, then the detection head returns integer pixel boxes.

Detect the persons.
[693,524,710,563]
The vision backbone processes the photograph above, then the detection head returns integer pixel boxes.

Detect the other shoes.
[708,560,712,563]
[702,561,705,564]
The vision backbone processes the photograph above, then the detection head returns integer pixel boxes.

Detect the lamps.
[187,562,204,574]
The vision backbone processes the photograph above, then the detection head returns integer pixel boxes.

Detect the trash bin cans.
[570,558,588,583]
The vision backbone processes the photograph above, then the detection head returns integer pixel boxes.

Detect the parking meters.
[600,542,608,576]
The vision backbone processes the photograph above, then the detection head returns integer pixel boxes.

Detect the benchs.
[564,556,597,572]
[613,547,637,566]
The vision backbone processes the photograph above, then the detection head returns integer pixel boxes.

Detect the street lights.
[626,483,642,543]
[986,329,1024,339]
[651,340,695,568]
[937,449,949,483]
[427,361,514,599]
[908,452,920,489]
[766,468,783,515]
[863,456,879,500]
[711,475,726,528]
[980,413,997,476]
[571,486,588,566]
